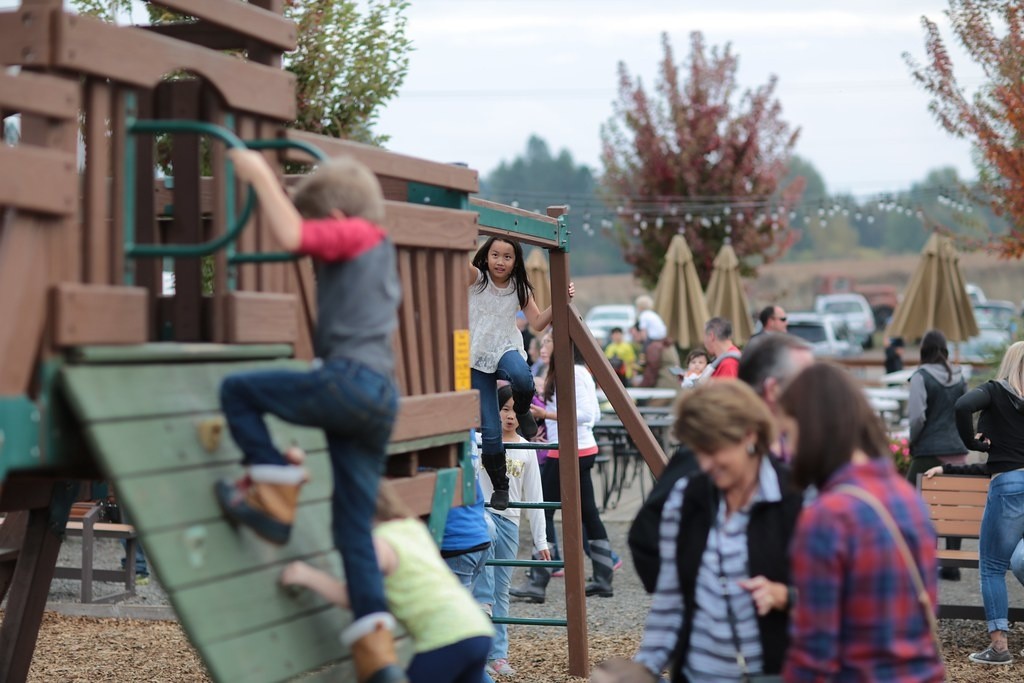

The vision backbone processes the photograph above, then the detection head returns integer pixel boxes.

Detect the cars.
[959,285,1017,355]
[585,305,638,347]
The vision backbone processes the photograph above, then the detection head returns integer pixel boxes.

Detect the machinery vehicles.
[824,272,899,324]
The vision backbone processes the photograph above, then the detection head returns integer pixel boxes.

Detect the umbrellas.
[646,233,717,347]
[884,231,979,346]
[705,242,754,343]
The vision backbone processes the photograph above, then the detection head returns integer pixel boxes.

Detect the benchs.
[916,472,1024,621]
[51,497,138,603]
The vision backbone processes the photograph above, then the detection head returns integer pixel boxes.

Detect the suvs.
[757,313,864,357]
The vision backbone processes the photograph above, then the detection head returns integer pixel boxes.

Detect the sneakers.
[969,647,1012,664]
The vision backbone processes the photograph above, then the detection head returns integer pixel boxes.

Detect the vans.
[815,294,876,342]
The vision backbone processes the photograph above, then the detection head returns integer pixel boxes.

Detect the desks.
[599,404,676,488]
[594,415,675,508]
[882,369,915,386]
[596,386,677,407]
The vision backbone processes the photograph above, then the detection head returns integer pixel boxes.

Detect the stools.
[596,439,639,513]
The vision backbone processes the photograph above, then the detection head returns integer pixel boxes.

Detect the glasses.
[780,317,787,321]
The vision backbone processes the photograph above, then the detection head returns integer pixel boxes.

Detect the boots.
[584,539,614,597]
[215,464,307,547]
[339,611,409,683]
[509,543,556,603]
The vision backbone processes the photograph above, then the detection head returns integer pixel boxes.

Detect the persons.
[681,349,715,385]
[116,519,149,585]
[603,295,681,388]
[281,471,494,683]
[883,338,903,388]
[906,331,967,581]
[955,340,1024,665]
[705,317,741,379]
[774,365,949,683]
[749,306,788,340]
[591,377,800,683]
[218,148,404,683]
[515,313,556,384]
[739,331,820,508]
[510,325,614,601]
[468,235,538,509]
[471,383,550,676]
[416,426,494,621]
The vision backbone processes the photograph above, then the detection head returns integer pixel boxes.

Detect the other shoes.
[524,569,565,578]
[486,658,516,676]
[938,567,961,581]
[105,566,150,586]
[585,560,623,582]
